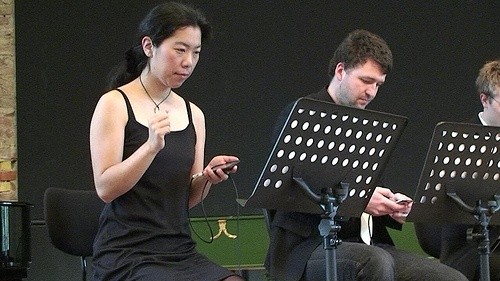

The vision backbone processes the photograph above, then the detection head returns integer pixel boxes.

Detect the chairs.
[44,187,104,281]
[413,221,441,258]
[264,209,275,235]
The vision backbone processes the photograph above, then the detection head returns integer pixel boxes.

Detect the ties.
[359,211,373,246]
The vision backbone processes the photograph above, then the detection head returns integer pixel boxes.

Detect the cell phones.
[397,199,413,205]
[211,160,240,173]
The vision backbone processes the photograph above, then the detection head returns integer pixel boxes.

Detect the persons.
[265,29,472,281]
[88,1,242,281]
[439,60,500,281]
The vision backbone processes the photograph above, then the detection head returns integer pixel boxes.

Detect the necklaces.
[139,74,171,115]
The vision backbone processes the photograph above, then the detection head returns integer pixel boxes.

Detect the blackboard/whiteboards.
[14,0,500,224]
